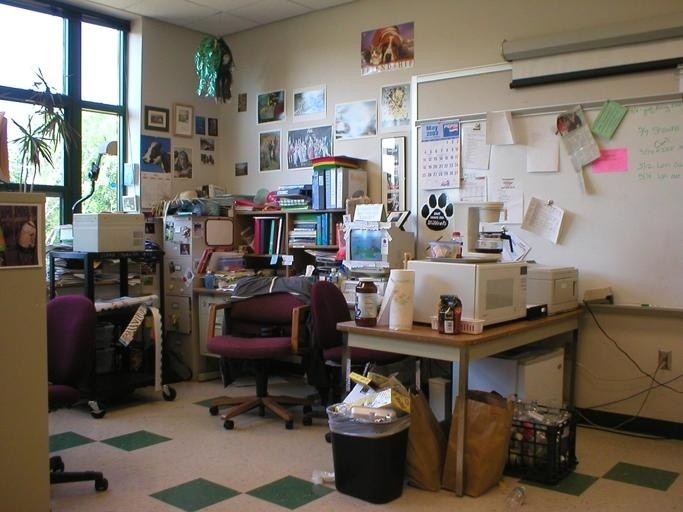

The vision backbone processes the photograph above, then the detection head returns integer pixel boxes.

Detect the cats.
[370,43,383,65]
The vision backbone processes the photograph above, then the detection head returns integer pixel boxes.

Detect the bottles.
[503,485,527,509]
[439,293,461,334]
[330,268,336,278]
[353,277,378,326]
[205,269,214,288]
[450,230,462,259]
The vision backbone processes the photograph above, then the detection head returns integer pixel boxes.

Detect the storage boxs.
[71,212,145,254]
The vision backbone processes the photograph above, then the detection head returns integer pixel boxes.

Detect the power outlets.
[656,347,672,371]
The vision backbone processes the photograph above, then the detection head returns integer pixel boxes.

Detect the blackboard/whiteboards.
[413,93,683,316]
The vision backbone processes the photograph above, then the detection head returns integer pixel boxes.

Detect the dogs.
[372,26,408,64]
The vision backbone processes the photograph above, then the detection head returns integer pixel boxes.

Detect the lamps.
[70,138,120,214]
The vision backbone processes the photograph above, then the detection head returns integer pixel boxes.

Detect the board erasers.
[584,287,613,304]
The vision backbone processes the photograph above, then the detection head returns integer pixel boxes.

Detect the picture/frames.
[171,103,194,139]
[386,210,411,230]
[144,105,169,133]
[379,135,406,218]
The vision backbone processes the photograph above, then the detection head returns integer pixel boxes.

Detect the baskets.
[504,400,578,485]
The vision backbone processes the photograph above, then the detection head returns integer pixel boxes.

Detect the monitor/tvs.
[345,221,415,281]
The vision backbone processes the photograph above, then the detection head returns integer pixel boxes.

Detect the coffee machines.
[453,201,514,263]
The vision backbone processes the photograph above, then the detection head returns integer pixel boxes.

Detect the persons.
[288,133,330,167]
[174,151,191,178]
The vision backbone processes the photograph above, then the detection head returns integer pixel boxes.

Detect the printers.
[519,260,579,316]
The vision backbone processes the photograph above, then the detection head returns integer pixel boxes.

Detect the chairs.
[300,280,420,443]
[46,292,111,495]
[204,273,318,435]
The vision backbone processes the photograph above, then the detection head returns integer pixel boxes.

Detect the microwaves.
[405,259,527,328]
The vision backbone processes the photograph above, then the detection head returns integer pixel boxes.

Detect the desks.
[191,285,432,391]
[0,191,51,510]
[335,305,584,498]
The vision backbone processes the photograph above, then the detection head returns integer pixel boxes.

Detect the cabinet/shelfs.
[161,213,236,382]
[48,247,178,420]
[232,204,346,276]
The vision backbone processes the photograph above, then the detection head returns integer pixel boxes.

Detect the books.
[241,165,368,284]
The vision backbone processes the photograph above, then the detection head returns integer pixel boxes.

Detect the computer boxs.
[342,281,388,309]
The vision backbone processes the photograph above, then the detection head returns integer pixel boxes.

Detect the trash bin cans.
[326,403,411,504]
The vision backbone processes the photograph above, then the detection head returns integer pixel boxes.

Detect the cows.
[142,141,171,173]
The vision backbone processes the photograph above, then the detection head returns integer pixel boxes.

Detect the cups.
[428,240,459,258]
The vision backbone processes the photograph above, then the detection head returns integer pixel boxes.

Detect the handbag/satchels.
[441,388,518,499]
[406,384,448,493]
